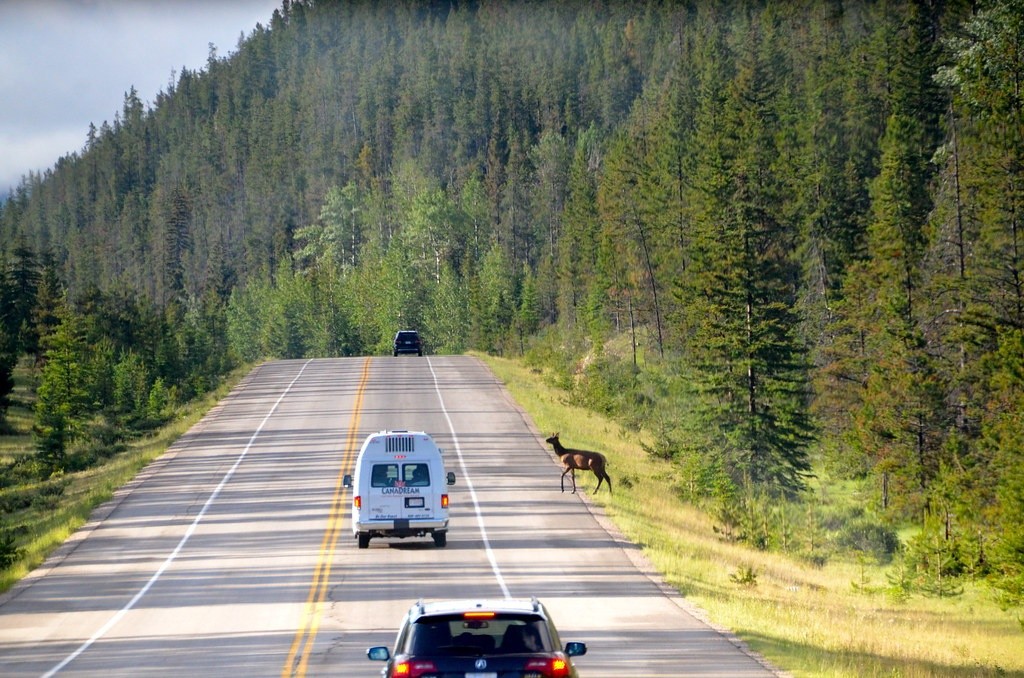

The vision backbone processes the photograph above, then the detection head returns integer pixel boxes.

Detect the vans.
[342,430,456,548]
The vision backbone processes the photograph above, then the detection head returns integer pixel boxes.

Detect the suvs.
[365,595,587,678]
[393,330,424,357]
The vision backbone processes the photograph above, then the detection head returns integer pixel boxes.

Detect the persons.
[412,469,417,480]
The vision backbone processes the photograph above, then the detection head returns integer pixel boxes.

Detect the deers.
[546,432,613,496]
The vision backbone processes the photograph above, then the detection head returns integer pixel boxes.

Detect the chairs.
[502,624,543,655]
[430,628,453,646]
